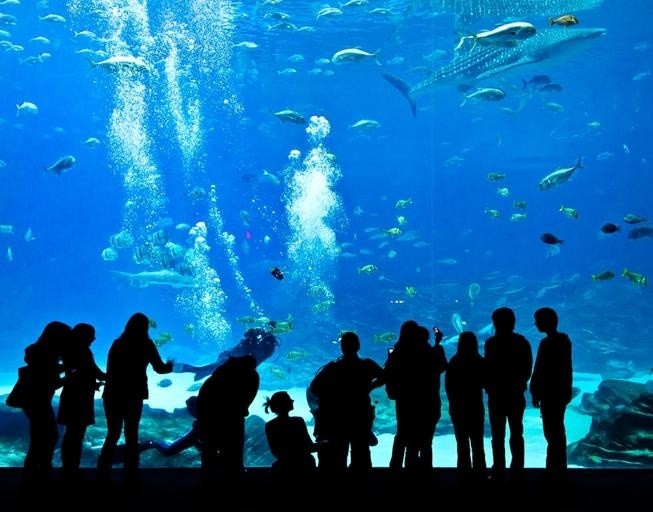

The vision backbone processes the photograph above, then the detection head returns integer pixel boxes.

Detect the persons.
[97,313,173,469]
[307,320,449,469]
[445,331,486,469]
[530,308,572,469]
[110,396,199,466]
[56,323,107,468]
[6,321,70,468]
[264,392,318,468]
[484,307,532,469]
[170,322,282,381]
[192,355,259,469]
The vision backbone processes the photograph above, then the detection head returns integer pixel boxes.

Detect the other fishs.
[0,0,392,78]
[97,152,652,392]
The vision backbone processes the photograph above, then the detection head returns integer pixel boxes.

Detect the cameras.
[388,348,393,353]
[433,326,439,333]
